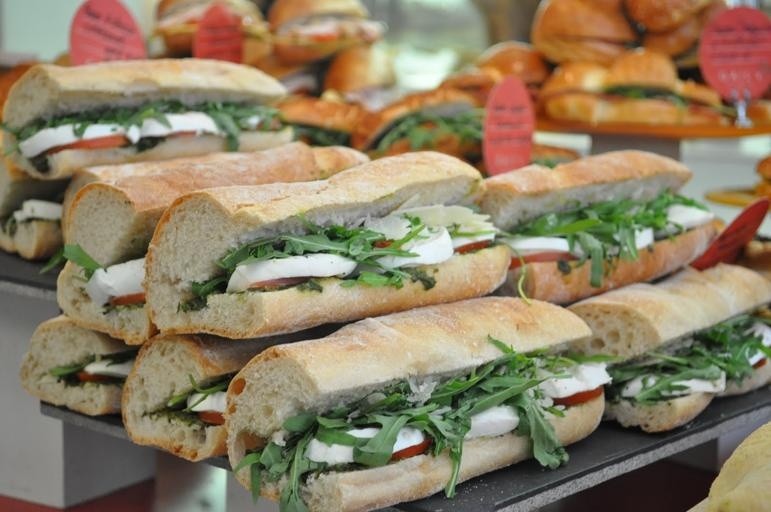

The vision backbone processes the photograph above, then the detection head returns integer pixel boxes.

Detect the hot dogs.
[0,0,771,512]
